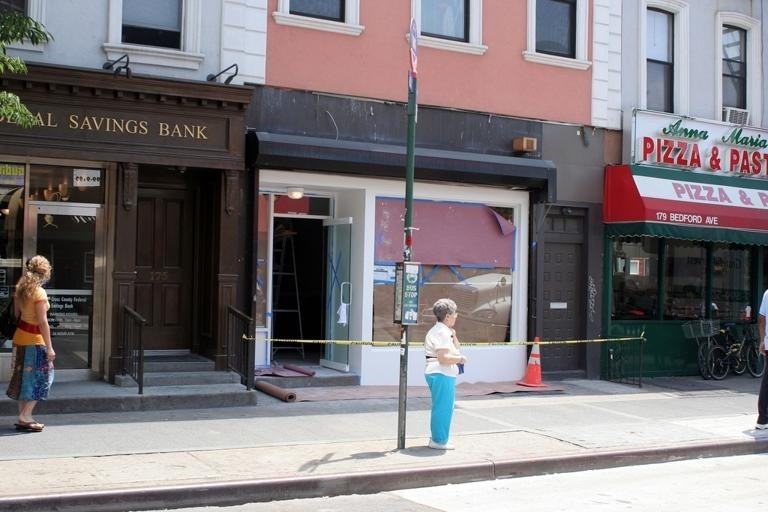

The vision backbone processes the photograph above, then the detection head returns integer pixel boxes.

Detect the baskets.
[700,316,721,339]
[682,320,700,339]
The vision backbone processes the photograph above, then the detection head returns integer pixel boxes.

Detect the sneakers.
[428,437,456,451]
[753,423,768,430]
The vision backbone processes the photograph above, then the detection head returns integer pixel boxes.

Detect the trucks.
[447,273,512,337]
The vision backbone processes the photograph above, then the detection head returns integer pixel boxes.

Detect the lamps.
[0,175,86,229]
[103,55,132,80]
[207,64,238,84]
[287,187,304,199]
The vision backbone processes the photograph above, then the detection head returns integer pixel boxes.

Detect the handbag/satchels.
[457,363,465,375]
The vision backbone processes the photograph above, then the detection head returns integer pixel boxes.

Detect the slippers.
[15,420,44,433]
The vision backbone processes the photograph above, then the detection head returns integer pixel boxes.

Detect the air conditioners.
[722,107,749,125]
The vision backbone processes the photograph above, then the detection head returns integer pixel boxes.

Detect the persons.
[6,253,55,432]
[755,289,768,430]
[422,297,467,450]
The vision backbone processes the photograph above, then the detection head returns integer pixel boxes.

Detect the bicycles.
[682,319,767,380]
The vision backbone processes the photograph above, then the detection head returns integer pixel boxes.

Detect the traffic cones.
[516,336,551,387]
